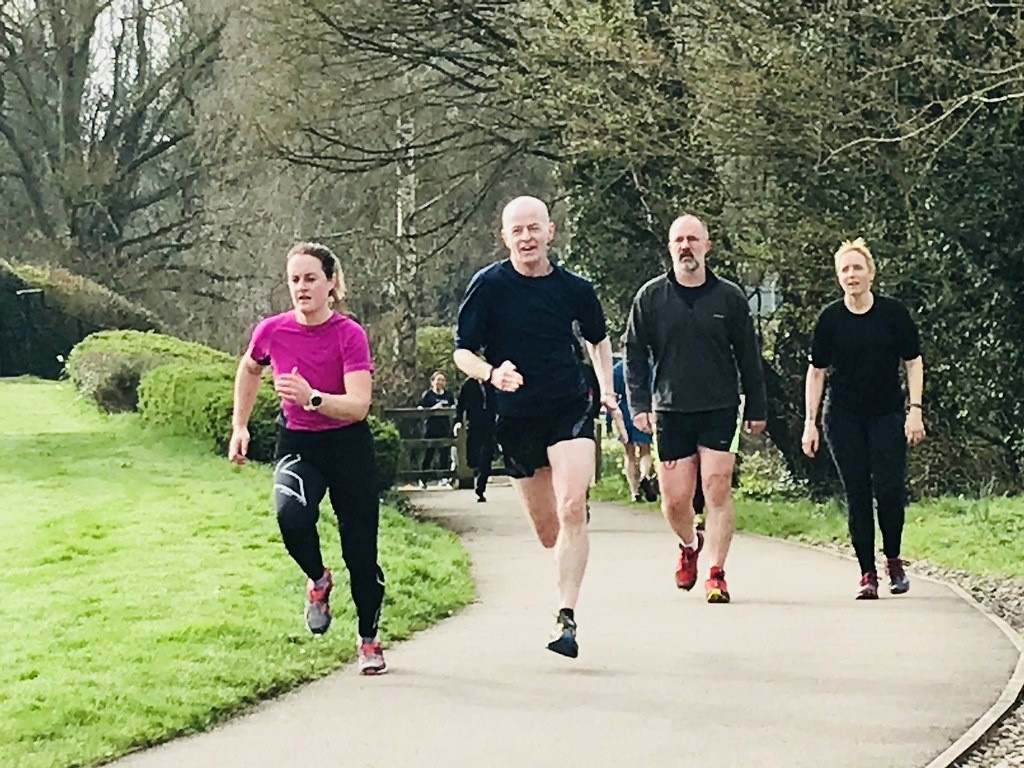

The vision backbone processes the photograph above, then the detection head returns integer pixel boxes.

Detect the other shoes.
[419,479,427,489]
[640,474,657,502]
[442,478,453,490]
[477,495,485,502]
[631,492,642,503]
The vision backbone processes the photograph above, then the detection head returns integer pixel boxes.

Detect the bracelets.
[600,392,619,398]
[906,403,925,412]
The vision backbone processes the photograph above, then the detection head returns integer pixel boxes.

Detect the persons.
[801,236,926,601]
[227,241,387,676]
[625,216,767,603]
[416,334,708,529]
[454,195,629,660]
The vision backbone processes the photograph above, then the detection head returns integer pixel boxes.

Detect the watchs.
[303,389,324,412]
[481,363,494,384]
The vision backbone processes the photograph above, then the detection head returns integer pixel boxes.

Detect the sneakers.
[304,568,333,636]
[546,612,578,659]
[676,532,703,591]
[884,558,911,592]
[356,635,387,675]
[856,572,878,599]
[705,579,730,603]
